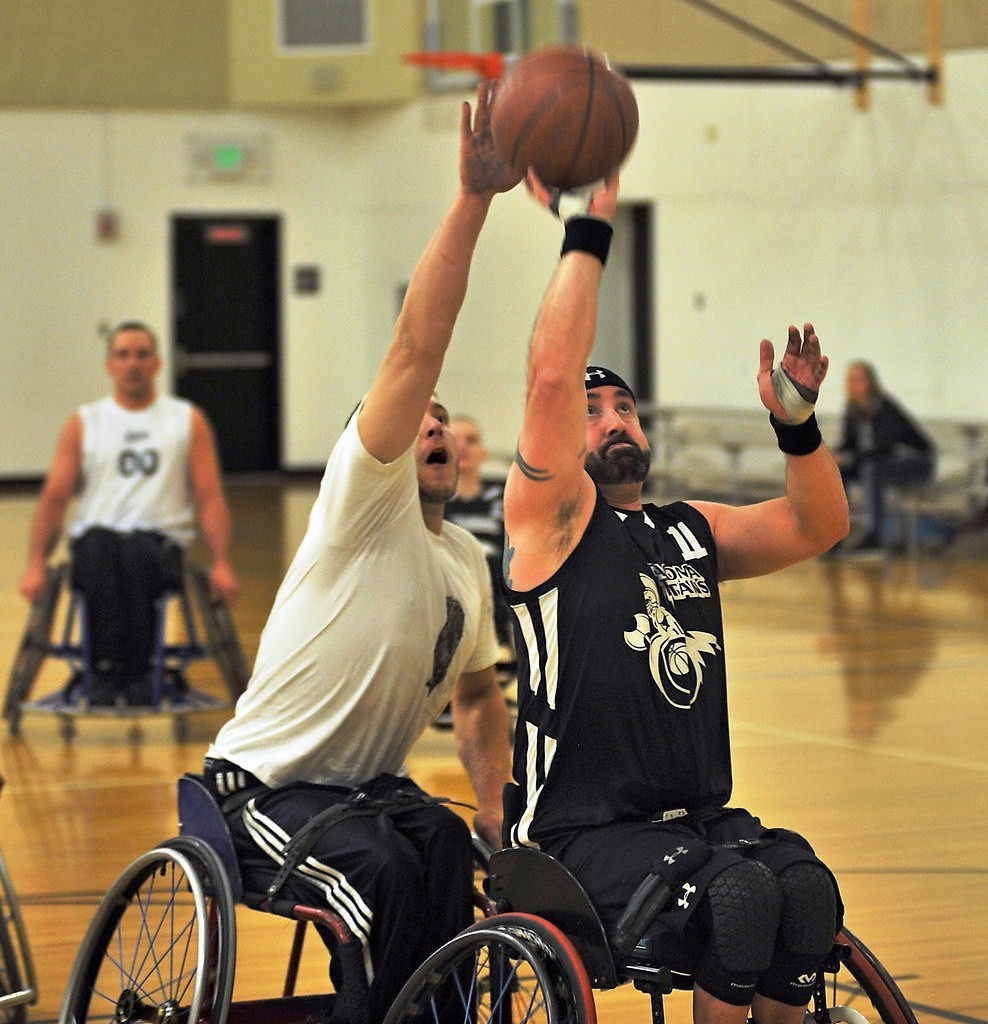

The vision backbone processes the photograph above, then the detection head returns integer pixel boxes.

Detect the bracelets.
[561,217,613,265]
[770,412,822,456]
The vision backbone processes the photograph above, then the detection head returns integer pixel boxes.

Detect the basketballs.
[490,46,638,188]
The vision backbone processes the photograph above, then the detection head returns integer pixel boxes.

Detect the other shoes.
[123,663,156,705]
[846,532,885,555]
[85,664,120,706]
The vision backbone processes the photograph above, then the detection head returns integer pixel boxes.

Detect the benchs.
[591,403,982,561]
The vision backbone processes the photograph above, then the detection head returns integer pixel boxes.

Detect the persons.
[201,79,513,1024]
[502,166,848,1024]
[821,362,934,558]
[446,415,507,638]
[18,323,241,706]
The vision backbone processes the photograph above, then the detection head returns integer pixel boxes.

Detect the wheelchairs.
[0,554,251,740]
[381,810,919,1024]
[69,772,485,1023]
